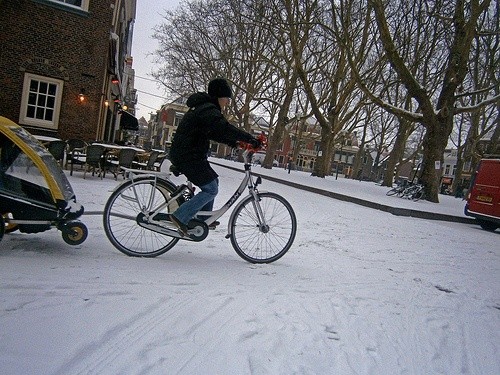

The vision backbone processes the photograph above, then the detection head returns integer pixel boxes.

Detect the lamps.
[111,92,121,103]
[103,100,108,107]
[123,104,127,110]
[78,92,84,103]
[109,70,119,83]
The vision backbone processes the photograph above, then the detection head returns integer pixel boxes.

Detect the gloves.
[251,137,261,149]
[234,142,239,153]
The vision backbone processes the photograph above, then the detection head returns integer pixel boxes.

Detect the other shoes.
[169,214,189,237]
[210,221,220,226]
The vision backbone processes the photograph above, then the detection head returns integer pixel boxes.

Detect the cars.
[251,153,280,167]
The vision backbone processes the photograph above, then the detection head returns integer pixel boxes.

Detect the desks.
[18,134,62,168]
[91,143,146,179]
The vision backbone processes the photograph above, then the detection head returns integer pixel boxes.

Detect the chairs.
[9,136,169,182]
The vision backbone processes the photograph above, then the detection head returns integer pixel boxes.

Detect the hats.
[208,79,231,98]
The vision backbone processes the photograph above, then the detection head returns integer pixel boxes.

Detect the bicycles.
[102,130,297,265]
[386,177,425,202]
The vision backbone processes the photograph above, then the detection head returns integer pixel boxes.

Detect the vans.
[463,154,500,232]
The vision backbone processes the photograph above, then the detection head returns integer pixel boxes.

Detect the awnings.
[121,110,139,131]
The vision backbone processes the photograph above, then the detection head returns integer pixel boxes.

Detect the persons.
[169,76,264,237]
